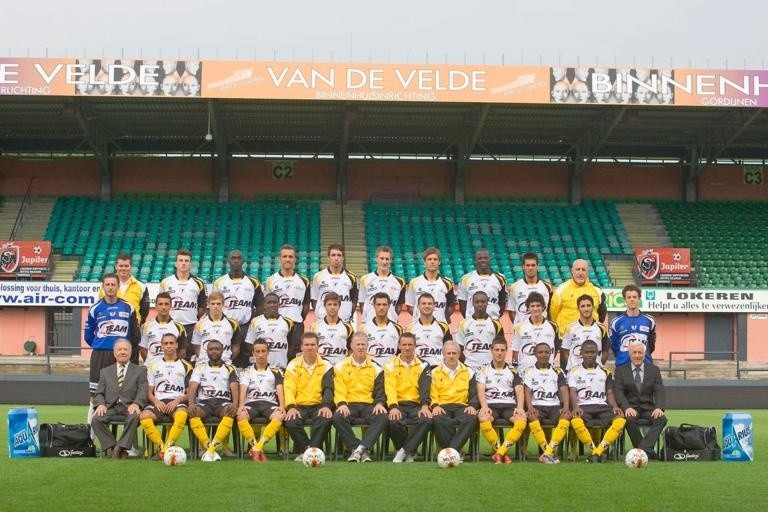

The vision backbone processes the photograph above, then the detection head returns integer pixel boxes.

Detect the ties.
[118,365,125,386]
[634,368,641,391]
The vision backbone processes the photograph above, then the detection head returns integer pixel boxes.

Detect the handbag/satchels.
[39,423,96,458]
[660,423,721,462]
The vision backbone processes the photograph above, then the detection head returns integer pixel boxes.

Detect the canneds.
[7,406,41,458]
[722,412,753,462]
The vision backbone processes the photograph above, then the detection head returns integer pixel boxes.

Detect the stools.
[97,404,674,467]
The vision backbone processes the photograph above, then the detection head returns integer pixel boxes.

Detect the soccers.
[303,447,326,465]
[626,448,647,467]
[164,446,187,465]
[437,448,461,468]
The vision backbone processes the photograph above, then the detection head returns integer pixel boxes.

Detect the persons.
[82,245,669,465]
[75,73,199,96]
[550,79,676,105]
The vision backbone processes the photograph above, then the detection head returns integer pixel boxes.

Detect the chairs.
[360,189,635,290]
[653,194,768,291]
[45,194,321,289]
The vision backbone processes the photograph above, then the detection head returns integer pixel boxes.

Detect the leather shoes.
[120,450,128,460]
[111,448,120,459]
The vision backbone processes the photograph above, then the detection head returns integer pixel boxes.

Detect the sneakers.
[248,448,372,462]
[539,450,615,464]
[152,452,164,461]
[212,452,221,461]
[220,448,236,456]
[392,448,512,464]
[201,450,213,461]
[126,447,139,456]
